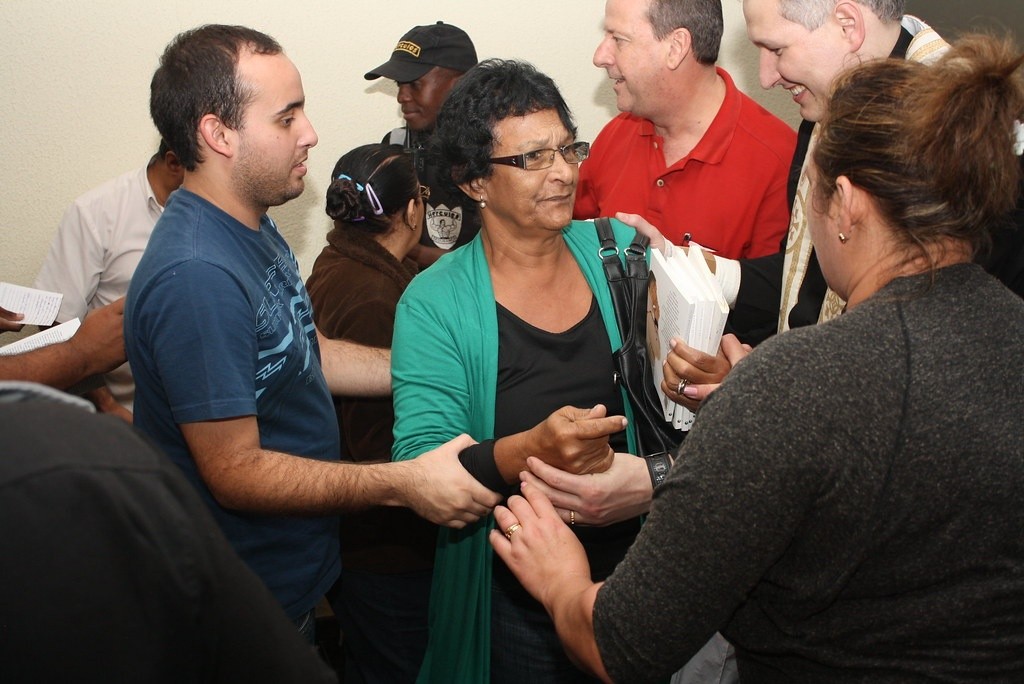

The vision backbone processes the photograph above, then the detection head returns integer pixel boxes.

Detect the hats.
[363,21,478,83]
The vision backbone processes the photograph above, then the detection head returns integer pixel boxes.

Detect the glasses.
[408,185,430,204]
[487,140,590,171]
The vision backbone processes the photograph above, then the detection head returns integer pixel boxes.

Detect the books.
[647,245,730,431]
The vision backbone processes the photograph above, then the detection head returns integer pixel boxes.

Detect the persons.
[391,58,734,684]
[616,0,1023,418]
[305,143,438,684]
[120,23,499,645]
[0,402,336,684]
[36,141,189,424]
[645,270,660,364]
[571,0,799,260]
[489,33,1024,683]
[365,21,479,266]
[0,295,126,385]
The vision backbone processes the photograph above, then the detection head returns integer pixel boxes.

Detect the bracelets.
[646,451,672,489]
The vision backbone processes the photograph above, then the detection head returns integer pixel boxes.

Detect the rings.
[678,379,686,394]
[570,511,575,524]
[505,524,520,538]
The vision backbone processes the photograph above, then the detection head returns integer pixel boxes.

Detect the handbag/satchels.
[593,213,679,457]
